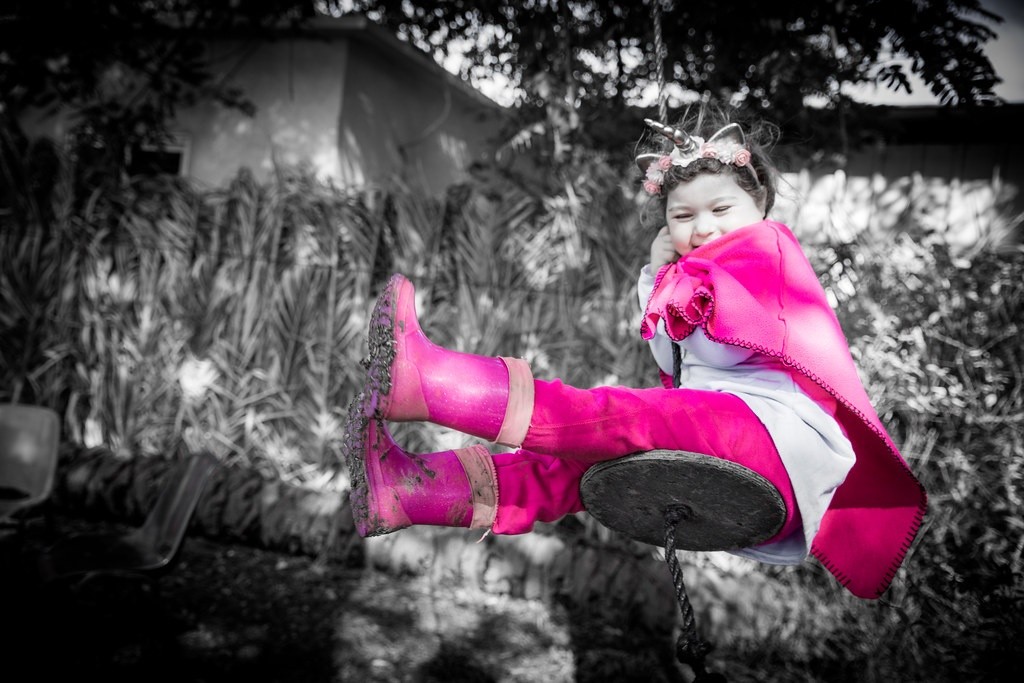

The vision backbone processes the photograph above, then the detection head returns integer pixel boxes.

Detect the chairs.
[0,403,60,524]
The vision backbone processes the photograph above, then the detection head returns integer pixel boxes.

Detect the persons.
[341,119,928,599]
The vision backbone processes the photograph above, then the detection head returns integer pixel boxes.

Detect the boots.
[363,273,534,448]
[343,391,499,538]
